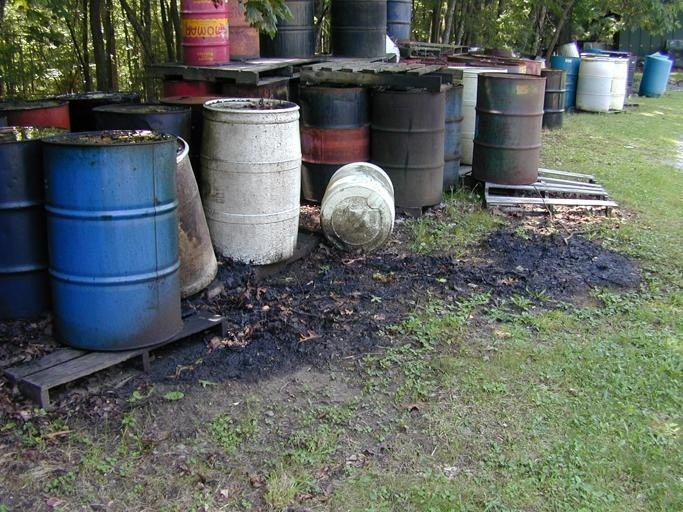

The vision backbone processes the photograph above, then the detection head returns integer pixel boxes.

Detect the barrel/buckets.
[639,54,673,98]
[0,0,635,351]
